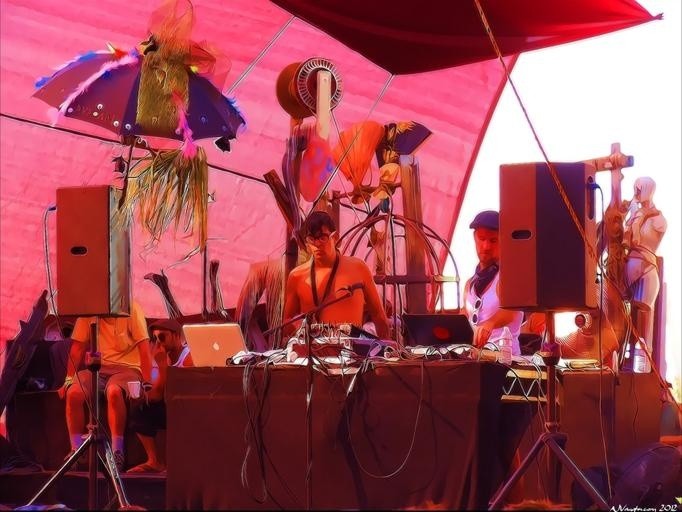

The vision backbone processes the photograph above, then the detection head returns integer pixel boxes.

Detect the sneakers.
[105,449,124,475]
[64,450,88,465]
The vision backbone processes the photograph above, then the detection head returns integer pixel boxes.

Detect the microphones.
[341,282,365,291]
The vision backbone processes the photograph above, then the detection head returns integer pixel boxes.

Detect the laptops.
[183,323,249,367]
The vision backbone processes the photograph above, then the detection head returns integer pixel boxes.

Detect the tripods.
[488,314,612,512]
[25,323,131,507]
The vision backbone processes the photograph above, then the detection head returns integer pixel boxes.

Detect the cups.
[127,381,140,399]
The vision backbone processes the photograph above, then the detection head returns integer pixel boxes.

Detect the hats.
[149,319,181,333]
[470,211,499,228]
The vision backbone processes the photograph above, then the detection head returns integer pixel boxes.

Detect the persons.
[463,210,524,354]
[58,302,194,475]
[281,211,390,349]
[622,177,668,311]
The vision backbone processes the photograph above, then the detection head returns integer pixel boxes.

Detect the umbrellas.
[30,51,245,210]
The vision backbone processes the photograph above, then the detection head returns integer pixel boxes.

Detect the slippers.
[126,464,164,475]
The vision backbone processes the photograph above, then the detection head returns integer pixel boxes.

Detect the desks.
[161,353,483,508]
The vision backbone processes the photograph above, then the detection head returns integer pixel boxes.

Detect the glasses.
[306,230,336,244]
[151,332,169,343]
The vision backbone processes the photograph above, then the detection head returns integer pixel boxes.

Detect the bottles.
[498,326,513,366]
[633,337,647,375]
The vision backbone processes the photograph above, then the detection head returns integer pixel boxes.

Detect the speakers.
[55,184,132,318]
[499,162,598,311]
